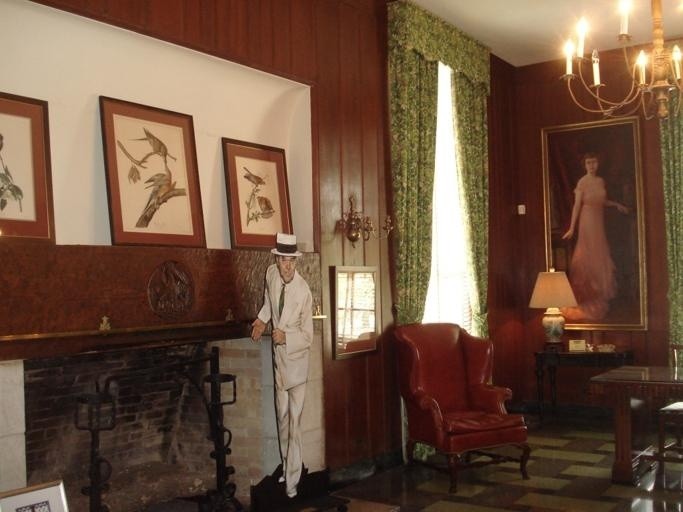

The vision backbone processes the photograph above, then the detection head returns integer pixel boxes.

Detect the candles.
[591,48,601,84]
[563,38,575,74]
[616,0,630,34]
[635,50,647,84]
[672,44,680,79]
[576,16,589,57]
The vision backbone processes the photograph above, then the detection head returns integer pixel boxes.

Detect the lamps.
[561,0,683,121]
[529,268,577,350]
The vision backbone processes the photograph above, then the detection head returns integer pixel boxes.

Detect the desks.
[588,365,683,484]
[536,351,631,424]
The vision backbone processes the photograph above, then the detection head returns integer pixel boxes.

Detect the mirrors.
[332,265,377,361]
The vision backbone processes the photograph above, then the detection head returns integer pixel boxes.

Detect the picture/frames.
[541,115,649,330]
[221,136,293,250]
[100,95,206,246]
[0,479,68,512]
[0,92,56,243]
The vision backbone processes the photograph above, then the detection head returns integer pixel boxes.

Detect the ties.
[278,283,286,317]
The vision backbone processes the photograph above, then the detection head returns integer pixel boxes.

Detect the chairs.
[655,343,683,471]
[387,322,531,494]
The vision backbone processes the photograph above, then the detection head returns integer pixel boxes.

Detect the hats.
[269,231,304,257]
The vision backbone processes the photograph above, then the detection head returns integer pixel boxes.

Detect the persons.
[559,149,632,323]
[248,231,315,497]
